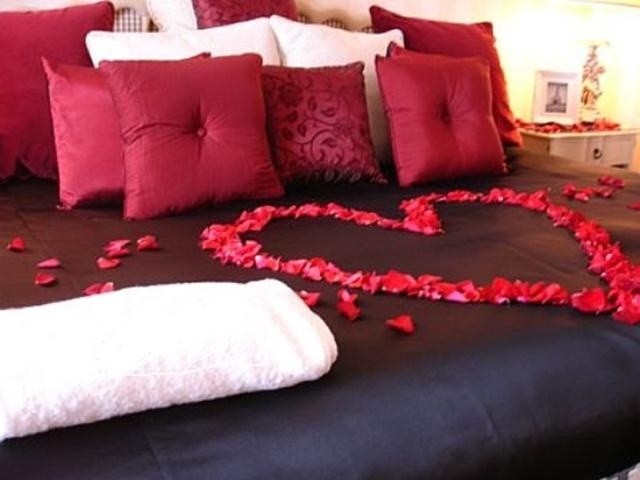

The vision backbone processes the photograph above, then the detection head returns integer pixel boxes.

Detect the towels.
[3,274,340,445]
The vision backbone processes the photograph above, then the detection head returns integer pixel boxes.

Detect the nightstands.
[517,123,640,169]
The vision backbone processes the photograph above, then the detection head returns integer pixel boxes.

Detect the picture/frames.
[531,68,582,129]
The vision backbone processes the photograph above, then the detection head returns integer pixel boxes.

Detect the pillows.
[82,14,282,68]
[271,13,415,155]
[370,44,512,184]
[1,1,118,185]
[190,1,301,30]
[146,2,199,33]
[100,54,279,222]
[367,5,526,154]
[253,55,384,186]
[36,48,130,214]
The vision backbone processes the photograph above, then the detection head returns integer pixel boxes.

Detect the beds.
[2,147,639,480]
